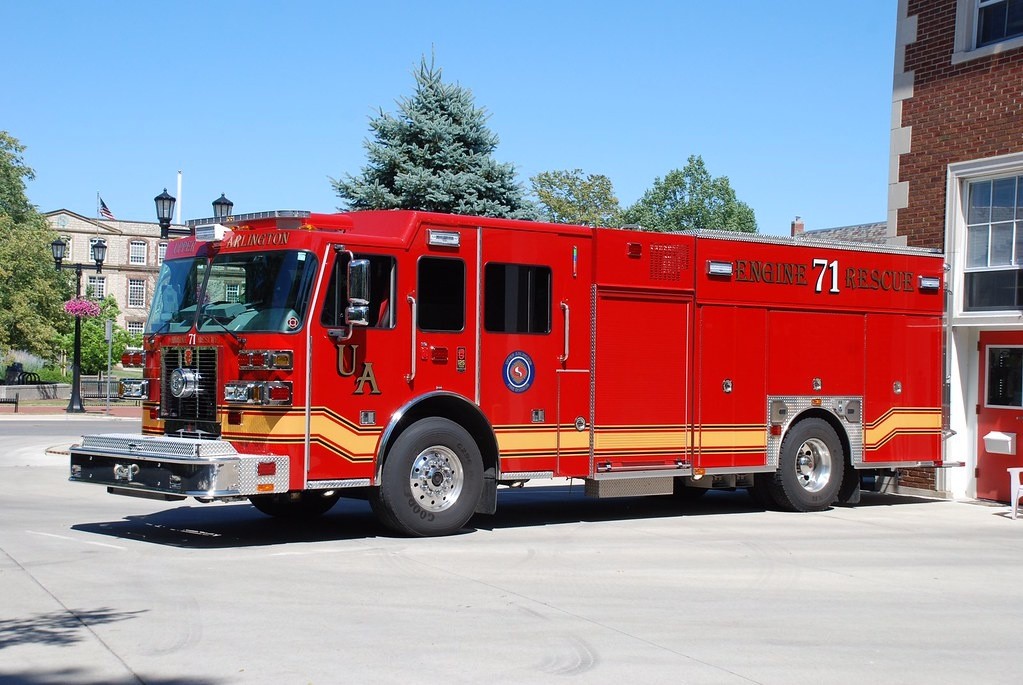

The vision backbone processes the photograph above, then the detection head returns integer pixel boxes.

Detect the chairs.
[1007,468,1023,520]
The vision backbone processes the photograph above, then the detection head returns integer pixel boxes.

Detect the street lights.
[52,237,109,412]
[154,188,233,322]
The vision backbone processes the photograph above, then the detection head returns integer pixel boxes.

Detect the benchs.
[0,393,19,413]
[81,381,141,407]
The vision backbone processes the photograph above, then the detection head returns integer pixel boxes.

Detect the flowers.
[64,299,103,317]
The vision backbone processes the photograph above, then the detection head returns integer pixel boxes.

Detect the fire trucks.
[68,209,967,539]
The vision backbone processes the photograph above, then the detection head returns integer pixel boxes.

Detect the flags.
[99,196,116,221]
